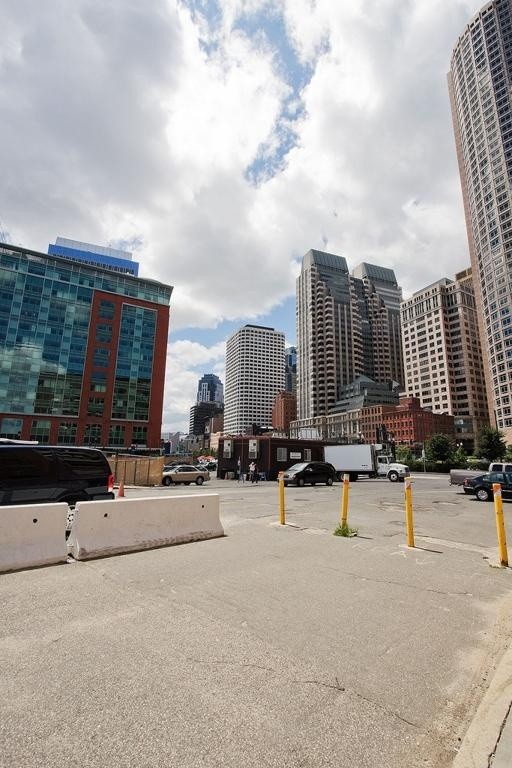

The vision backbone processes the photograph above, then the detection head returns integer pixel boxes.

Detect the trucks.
[323,443,410,482]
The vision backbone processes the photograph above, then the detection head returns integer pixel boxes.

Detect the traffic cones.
[117,479,125,497]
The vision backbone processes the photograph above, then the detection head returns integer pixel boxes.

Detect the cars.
[277,461,337,486]
[162,460,216,485]
[462,471,512,501]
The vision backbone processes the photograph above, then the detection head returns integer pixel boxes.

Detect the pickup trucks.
[449,462,512,494]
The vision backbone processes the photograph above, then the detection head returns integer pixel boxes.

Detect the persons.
[248,461,258,483]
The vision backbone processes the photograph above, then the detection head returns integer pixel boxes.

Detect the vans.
[0,443,115,536]
